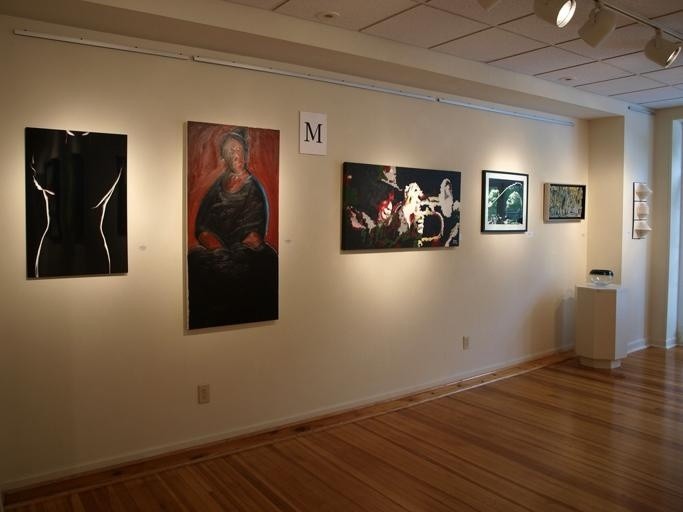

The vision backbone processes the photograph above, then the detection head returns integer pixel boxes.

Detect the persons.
[192,131,277,325]
[345,166,460,250]
[30,128,123,278]
[490,188,524,226]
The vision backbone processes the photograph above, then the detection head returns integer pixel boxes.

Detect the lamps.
[530,0,682,72]
[479,169,529,234]
